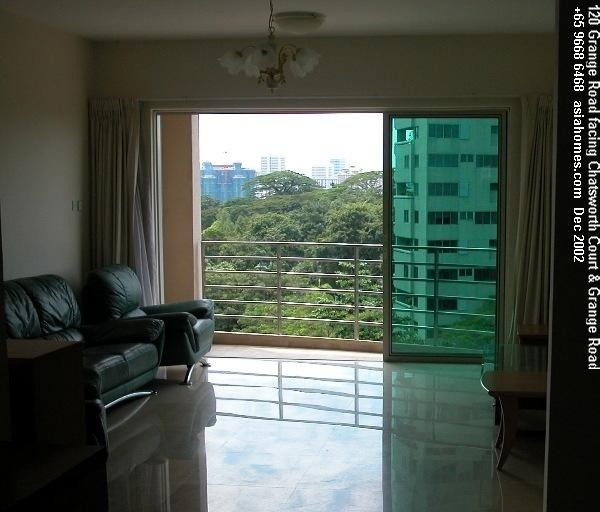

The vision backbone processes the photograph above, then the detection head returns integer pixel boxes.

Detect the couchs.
[4,274,166,410]
[81,263,215,384]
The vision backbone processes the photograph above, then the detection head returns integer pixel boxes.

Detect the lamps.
[217,0,322,93]
[275,12,325,36]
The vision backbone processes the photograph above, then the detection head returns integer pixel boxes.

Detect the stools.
[480,370,546,471]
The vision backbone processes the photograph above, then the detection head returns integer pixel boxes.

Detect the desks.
[519,325,548,345]
[0,443,109,511]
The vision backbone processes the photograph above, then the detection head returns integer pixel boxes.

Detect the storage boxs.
[7,339,85,448]
